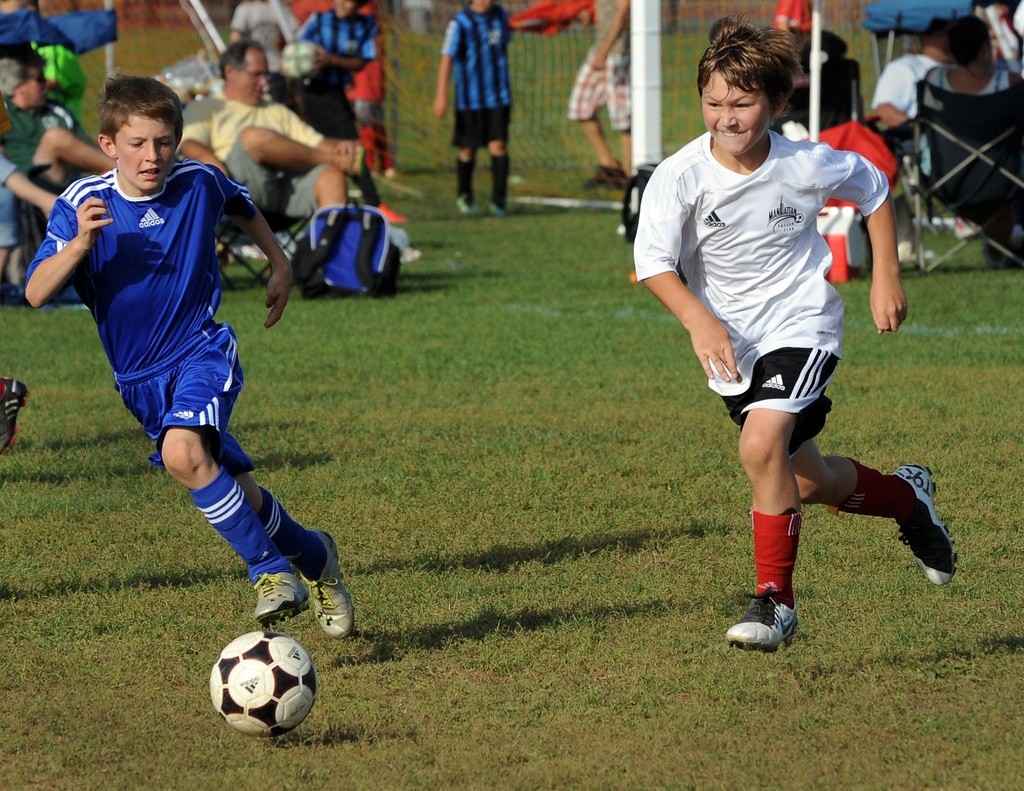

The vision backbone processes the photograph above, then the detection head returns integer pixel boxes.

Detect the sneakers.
[723,587,800,650]
[291,528,356,640]
[888,464,958,587]
[0,374,31,457]
[252,565,313,628]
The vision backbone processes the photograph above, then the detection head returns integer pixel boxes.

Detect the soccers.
[208,628,320,739]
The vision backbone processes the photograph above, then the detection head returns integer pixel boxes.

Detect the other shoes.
[370,199,411,224]
[578,158,630,193]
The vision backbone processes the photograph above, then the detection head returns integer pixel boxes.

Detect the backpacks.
[292,198,402,300]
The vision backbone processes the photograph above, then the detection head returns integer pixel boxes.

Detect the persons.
[178,40,365,232]
[229,0,410,221]
[432,0,513,215]
[26,74,356,639]
[871,1,1024,238]
[568,0,631,189]
[774,0,810,32]
[0,0,117,282]
[786,30,933,260]
[633,25,957,651]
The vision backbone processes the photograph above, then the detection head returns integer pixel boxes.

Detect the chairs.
[862,82,1024,277]
[213,205,312,286]
